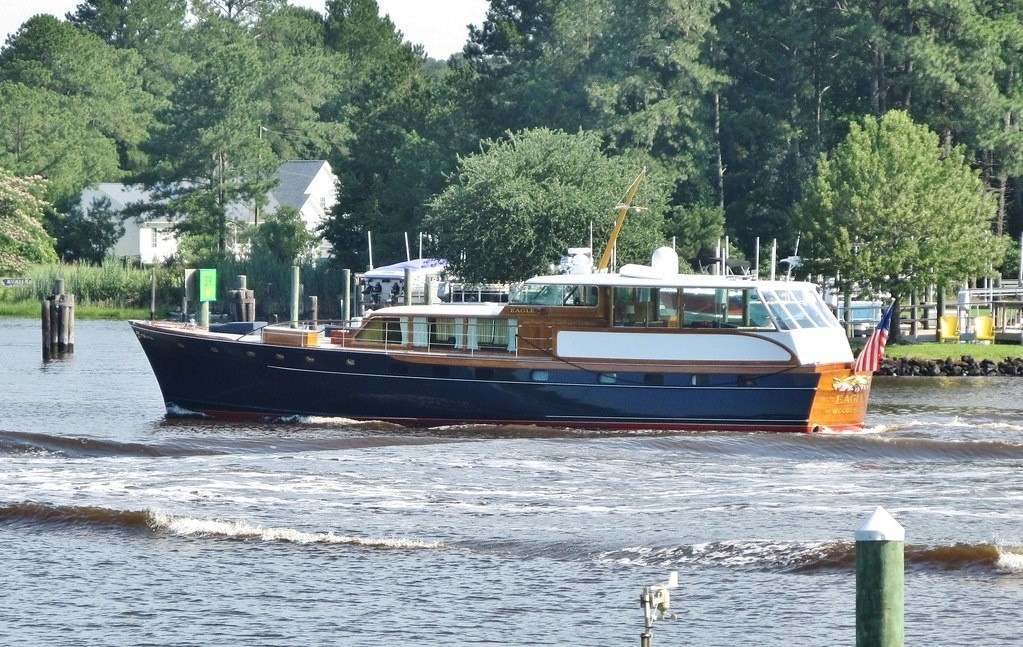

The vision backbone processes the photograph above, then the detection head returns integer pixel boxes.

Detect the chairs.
[974,316,996,345]
[937,315,960,344]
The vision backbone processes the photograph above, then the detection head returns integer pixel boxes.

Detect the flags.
[850,299,896,376]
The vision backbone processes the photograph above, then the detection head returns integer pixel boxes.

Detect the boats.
[126,167,881,438]
